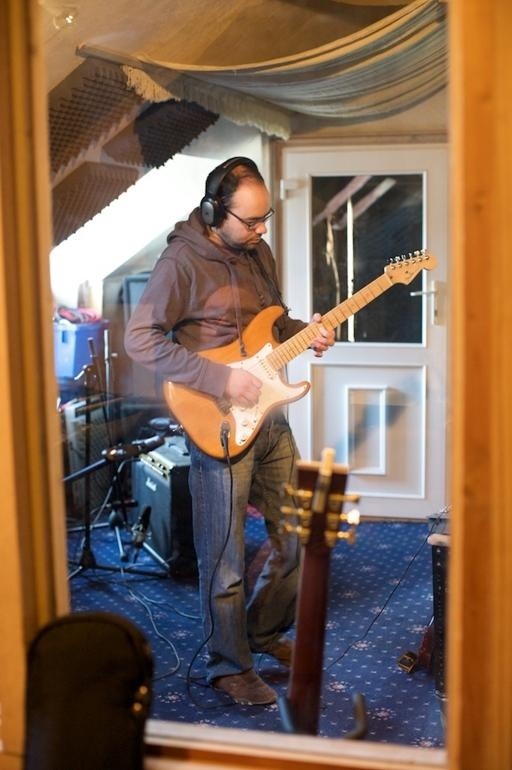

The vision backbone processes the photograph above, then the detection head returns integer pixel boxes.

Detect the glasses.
[223,205,275,232]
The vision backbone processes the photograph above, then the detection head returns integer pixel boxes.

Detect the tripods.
[64,337,167,583]
[67,329,143,561]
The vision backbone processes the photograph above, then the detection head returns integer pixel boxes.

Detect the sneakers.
[212,668,277,705]
[251,635,293,668]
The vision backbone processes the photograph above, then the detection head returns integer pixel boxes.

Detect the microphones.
[131,505,151,563]
[132,435,164,455]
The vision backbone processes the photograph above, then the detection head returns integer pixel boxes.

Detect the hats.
[23,612,155,769]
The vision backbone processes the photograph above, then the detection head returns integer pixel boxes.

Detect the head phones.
[201,157,258,226]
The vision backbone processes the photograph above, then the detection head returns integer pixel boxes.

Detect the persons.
[122,157,338,708]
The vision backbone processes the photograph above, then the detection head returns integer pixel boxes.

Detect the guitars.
[279,448,360,734]
[162,249,437,460]
